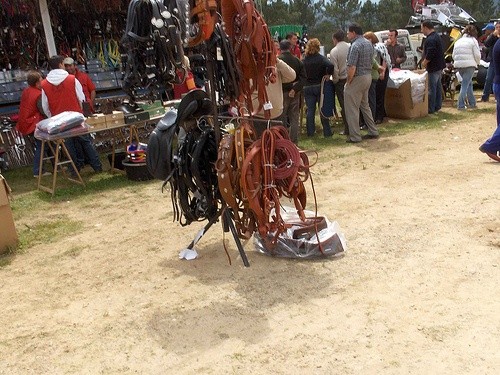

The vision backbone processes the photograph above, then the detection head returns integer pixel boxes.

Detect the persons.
[40,55,105,178]
[452,24,481,110]
[60,57,96,114]
[363,28,407,123]
[340,22,383,144]
[329,28,366,136]
[417,20,447,114]
[248,57,297,137]
[17,70,62,177]
[270,31,311,60]
[276,38,335,146]
[479,23,500,163]
[270,38,281,57]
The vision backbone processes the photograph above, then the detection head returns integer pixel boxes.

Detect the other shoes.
[479,145,500,162]
[360,125,368,130]
[476,98,489,103]
[345,139,362,143]
[374,120,383,125]
[339,131,349,135]
[57,167,68,172]
[33,172,52,177]
[361,135,379,139]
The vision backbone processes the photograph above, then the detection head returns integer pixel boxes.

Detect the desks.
[37,115,164,196]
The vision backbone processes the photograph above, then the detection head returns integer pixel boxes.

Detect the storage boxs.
[385,71,428,119]
[0,174,19,254]
[87,110,150,131]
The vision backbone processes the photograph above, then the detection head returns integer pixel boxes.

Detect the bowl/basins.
[107,153,127,171]
[121,160,151,181]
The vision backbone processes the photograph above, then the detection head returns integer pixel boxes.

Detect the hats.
[63,57,74,64]
[481,23,495,31]
